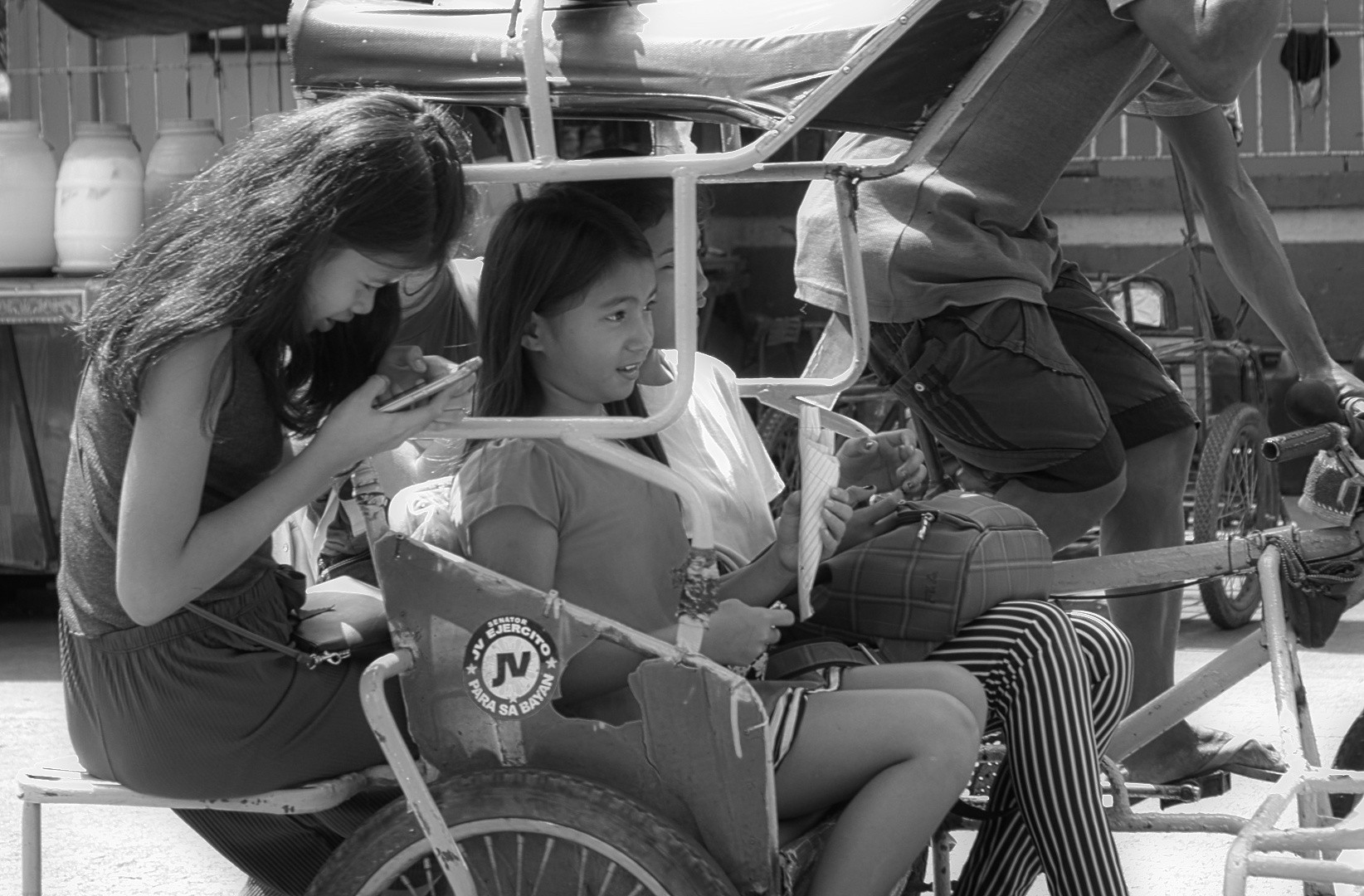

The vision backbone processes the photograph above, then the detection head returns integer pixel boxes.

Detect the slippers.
[1126,735,1287,805]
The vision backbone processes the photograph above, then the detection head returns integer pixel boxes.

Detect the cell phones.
[372,356,484,413]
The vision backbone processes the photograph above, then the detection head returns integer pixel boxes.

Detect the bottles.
[53,121,145,275]
[144,118,224,216]
[0,119,57,274]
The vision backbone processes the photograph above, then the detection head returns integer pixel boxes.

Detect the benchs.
[372,476,911,896]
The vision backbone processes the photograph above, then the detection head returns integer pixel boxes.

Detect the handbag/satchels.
[748,491,1053,678]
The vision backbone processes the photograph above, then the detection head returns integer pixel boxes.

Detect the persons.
[49,84,473,894]
[567,157,1134,896]
[460,196,986,896]
[791,0,1363,789]
